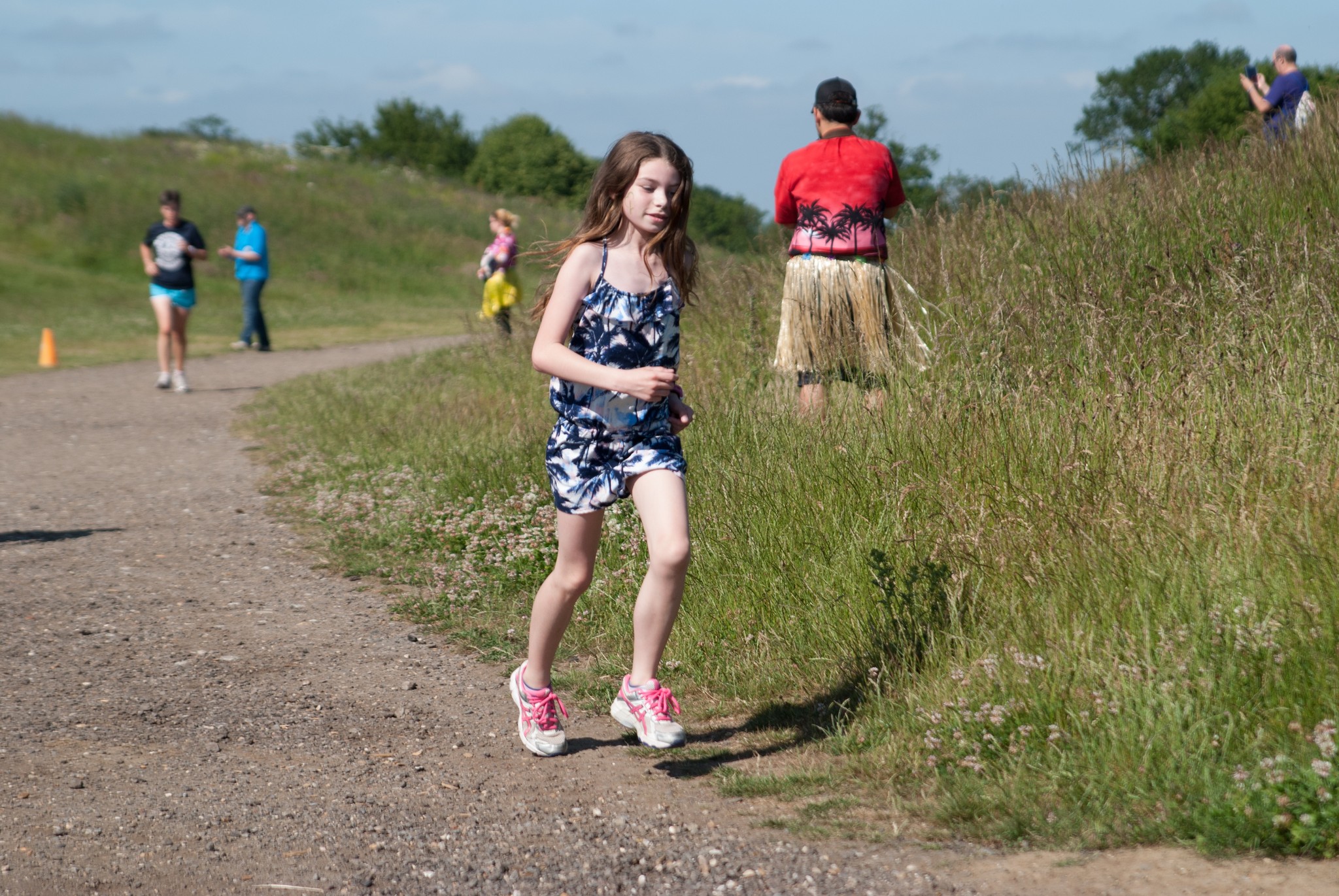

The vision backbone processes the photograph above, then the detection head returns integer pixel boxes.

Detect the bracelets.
[1248,86,1253,94]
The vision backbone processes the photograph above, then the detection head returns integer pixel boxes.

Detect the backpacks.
[1284,75,1316,131]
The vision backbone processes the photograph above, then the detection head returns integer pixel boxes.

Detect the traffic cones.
[36,327,60,368]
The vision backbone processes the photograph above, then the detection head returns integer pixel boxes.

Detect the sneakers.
[509,658,568,756]
[610,673,687,748]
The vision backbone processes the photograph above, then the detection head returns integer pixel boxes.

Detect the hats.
[811,78,857,113]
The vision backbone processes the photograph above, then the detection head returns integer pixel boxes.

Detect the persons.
[510,132,700,758]
[769,77,906,416]
[476,210,521,335]
[1239,42,1311,143]
[218,203,271,353]
[140,189,210,395]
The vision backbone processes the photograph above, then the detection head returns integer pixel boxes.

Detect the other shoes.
[230,340,250,351]
[251,341,261,350]
[172,374,189,392]
[159,371,171,388]
